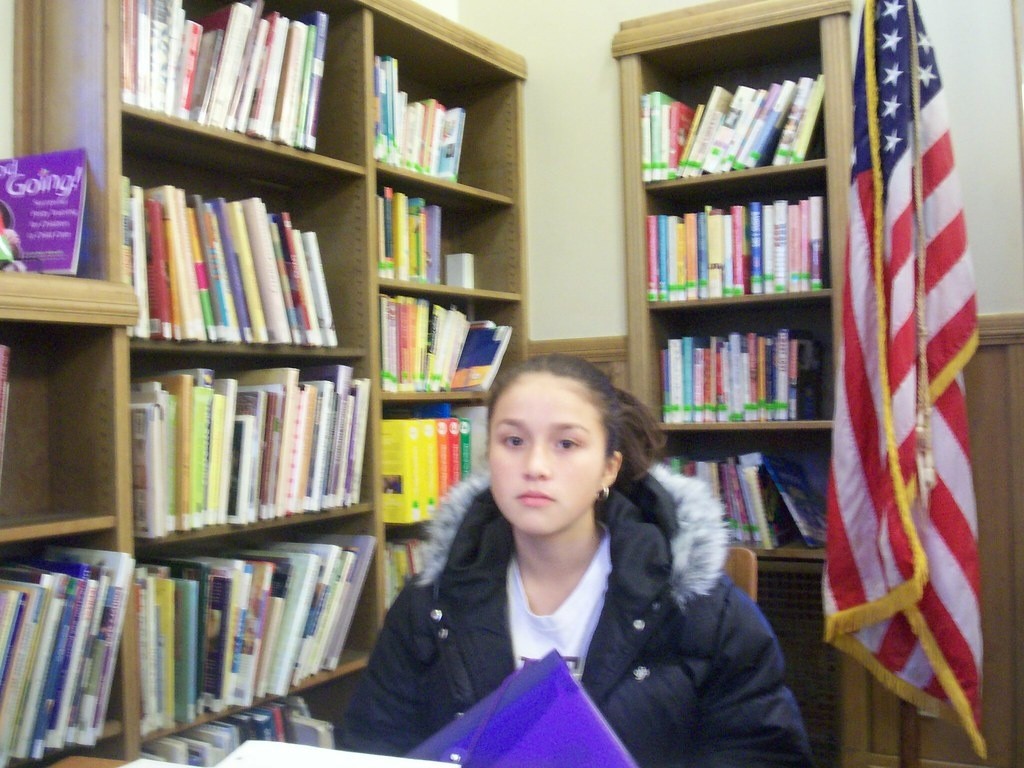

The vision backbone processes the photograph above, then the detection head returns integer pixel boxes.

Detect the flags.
[821,0,991,762]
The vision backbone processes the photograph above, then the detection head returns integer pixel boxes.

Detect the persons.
[337,353,809,768]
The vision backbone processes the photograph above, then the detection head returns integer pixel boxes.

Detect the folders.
[404,649,639,767]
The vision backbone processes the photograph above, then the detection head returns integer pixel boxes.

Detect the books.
[122,0,329,150]
[374,187,475,292]
[0,536,376,768]
[127,173,337,347]
[134,363,370,539]
[379,294,513,392]
[0,148,91,278]
[661,449,830,550]
[0,344,13,487]
[646,195,825,303]
[374,56,466,175]
[379,401,489,611]
[641,74,826,183]
[660,328,819,422]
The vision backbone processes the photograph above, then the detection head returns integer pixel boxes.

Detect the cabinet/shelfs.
[611,0,901,767]
[0,0,528,768]
